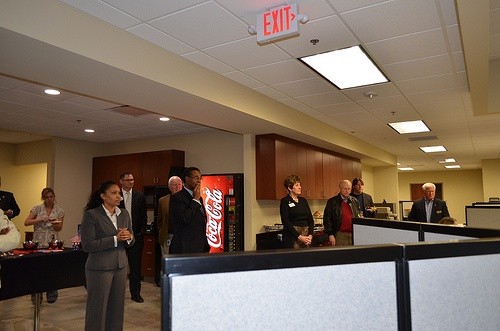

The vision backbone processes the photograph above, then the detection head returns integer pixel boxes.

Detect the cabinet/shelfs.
[92,150,185,282]
[256,133,362,200]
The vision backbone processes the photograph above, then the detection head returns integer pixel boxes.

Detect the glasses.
[123,179,135,182]
[189,176,204,181]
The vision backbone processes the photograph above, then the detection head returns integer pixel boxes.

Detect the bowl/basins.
[49,239,65,249]
[22,241,39,250]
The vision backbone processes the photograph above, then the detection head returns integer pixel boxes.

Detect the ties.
[126,192,132,226]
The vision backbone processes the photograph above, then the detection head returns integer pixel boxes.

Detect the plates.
[51,220,62,223]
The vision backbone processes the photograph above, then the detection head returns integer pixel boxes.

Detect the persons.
[81,180,136,331]
[280,175,321,249]
[25,188,65,303]
[409,182,458,224]
[0,176,20,220]
[158,167,210,255]
[118,173,147,302]
[323,178,375,247]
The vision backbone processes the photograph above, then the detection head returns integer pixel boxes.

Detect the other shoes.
[131,295,144,303]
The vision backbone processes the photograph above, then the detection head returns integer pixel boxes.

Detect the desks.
[0,247,88,331]
